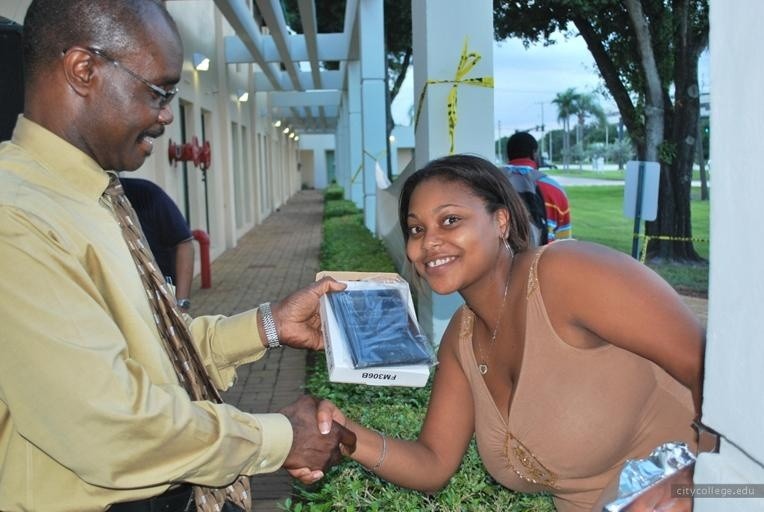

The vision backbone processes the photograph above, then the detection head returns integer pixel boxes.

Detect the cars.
[537,163,558,170]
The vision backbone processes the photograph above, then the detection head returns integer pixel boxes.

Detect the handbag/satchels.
[604,437,697,512]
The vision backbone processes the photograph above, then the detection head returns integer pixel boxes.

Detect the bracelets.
[358,426,387,472]
[257,301,284,350]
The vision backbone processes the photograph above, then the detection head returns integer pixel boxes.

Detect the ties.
[102,172,253,512]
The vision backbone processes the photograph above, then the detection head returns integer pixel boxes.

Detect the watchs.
[176,298,190,309]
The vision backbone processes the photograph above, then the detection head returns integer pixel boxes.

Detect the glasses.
[62,47,179,106]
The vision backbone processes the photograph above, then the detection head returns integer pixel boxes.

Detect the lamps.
[272,118,299,141]
[236,89,248,101]
[193,53,210,71]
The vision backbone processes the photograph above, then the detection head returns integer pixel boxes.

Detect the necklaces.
[470,262,515,374]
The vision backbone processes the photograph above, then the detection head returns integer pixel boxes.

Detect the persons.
[495,131,571,246]
[115,176,196,315]
[282,154,706,512]
[0,0,358,511]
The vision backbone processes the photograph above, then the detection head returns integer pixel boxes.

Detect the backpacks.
[501,168,548,246]
